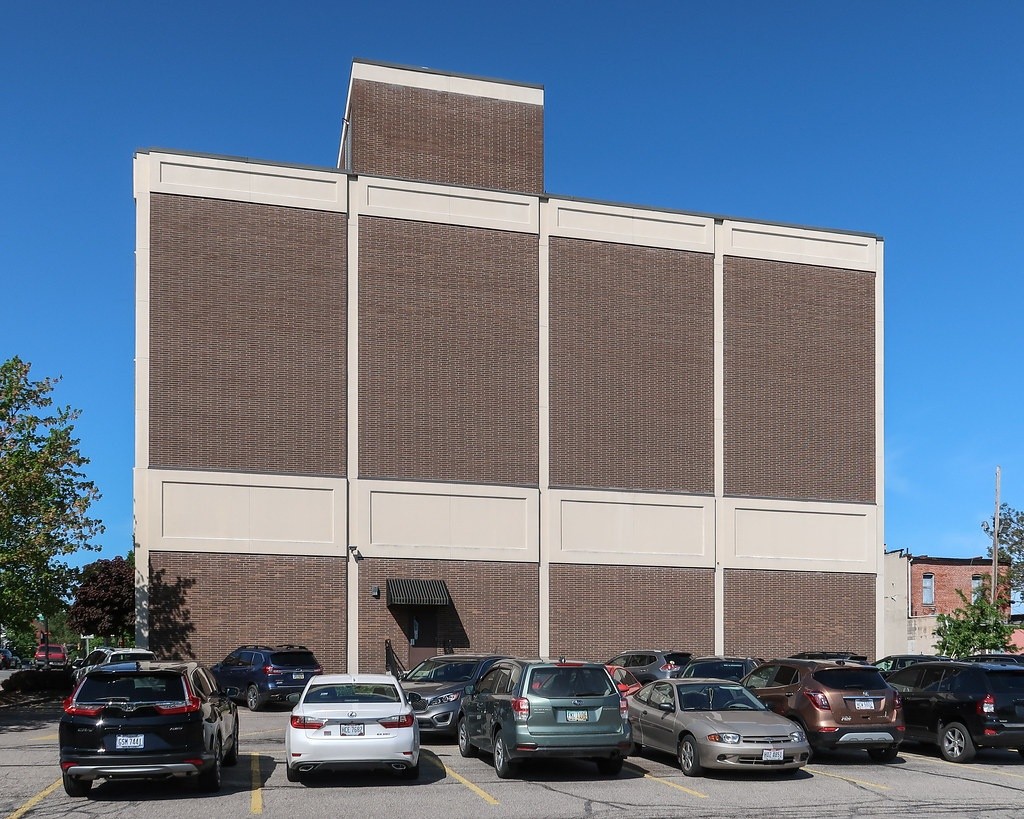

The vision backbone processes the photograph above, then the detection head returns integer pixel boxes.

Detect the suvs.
[394,654,518,738]
[882,659,1023,764]
[0,649,12,670]
[35,644,69,670]
[736,657,907,761]
[602,649,697,684]
[787,651,870,663]
[207,644,325,709]
[68,648,159,693]
[459,658,637,771]
[957,653,1024,665]
[678,655,766,687]
[870,654,964,680]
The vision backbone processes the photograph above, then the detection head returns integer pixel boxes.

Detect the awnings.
[386,578,450,607]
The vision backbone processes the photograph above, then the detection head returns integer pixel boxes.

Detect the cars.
[70,659,84,671]
[624,676,812,775]
[536,665,643,719]
[8,657,23,668]
[57,661,239,797]
[285,675,423,779]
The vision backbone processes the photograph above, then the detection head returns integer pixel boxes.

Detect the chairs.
[372,688,386,696]
[552,675,566,690]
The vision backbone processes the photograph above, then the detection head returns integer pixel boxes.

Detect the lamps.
[372,587,378,595]
[348,546,357,551]
